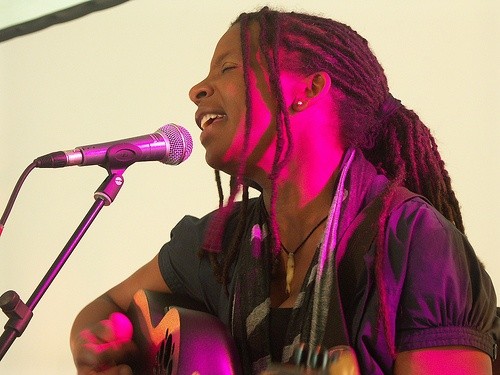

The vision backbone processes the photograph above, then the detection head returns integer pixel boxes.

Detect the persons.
[68,5,500,375]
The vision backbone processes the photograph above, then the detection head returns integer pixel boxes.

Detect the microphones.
[34,122,194,169]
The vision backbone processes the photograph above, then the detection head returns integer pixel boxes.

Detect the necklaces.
[279,215,329,295]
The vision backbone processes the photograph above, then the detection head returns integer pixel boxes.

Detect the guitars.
[122,287,363,375]
